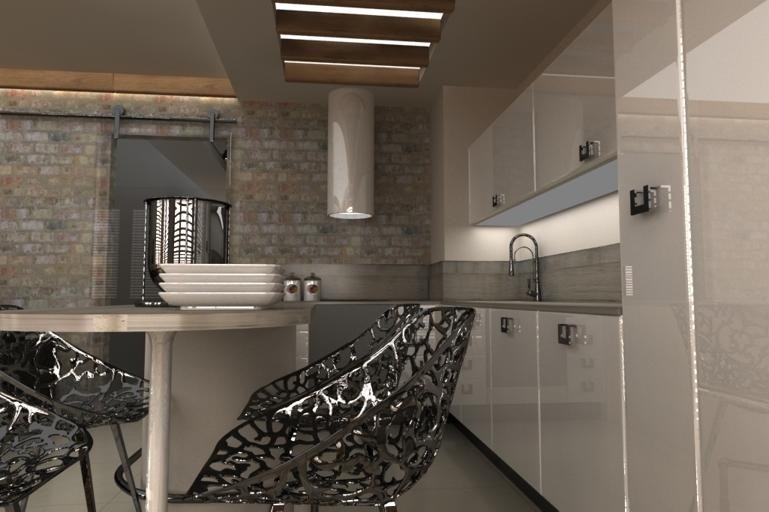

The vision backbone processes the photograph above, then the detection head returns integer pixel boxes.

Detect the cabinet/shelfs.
[447,300,622,501]
[467,5,616,229]
[612,1,701,512]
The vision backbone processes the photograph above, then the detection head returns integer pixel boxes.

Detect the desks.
[0,305,317,512]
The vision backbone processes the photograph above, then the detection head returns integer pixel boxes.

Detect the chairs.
[0,303,150,512]
[0,389,96,512]
[184,305,475,512]
[234,302,424,423]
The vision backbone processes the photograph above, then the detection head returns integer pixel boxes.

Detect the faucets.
[508,233,542,301]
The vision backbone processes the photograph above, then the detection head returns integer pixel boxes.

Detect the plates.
[158,292,285,305]
[159,282,283,291]
[159,262,283,273]
[158,273,283,282]
